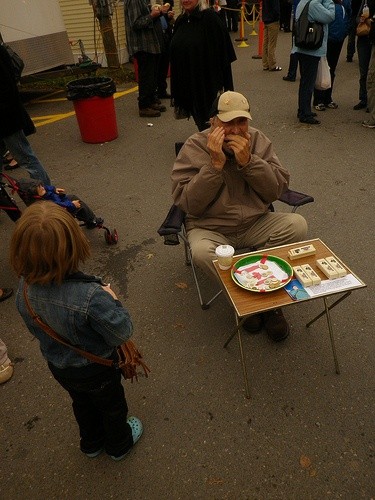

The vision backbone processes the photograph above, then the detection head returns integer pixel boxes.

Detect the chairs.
[156,141,315,311]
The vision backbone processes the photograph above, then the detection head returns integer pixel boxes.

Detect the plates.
[231,254,293,292]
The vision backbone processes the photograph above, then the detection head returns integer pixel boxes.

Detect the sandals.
[112,416,143,461]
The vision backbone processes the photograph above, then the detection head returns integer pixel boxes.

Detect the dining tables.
[212,238,367,399]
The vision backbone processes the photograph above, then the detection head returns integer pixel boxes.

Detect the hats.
[212,90,252,123]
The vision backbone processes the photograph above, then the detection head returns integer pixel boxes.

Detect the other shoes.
[283,76,296,81]
[139,104,166,117]
[297,112,317,118]
[2,154,20,170]
[0,288,14,303]
[158,94,172,99]
[242,310,289,342]
[313,103,325,111]
[299,117,320,125]
[87,217,105,230]
[327,102,338,109]
[353,101,367,110]
[269,66,282,71]
[361,120,375,129]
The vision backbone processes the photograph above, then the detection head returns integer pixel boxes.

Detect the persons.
[225,0,239,32]
[171,91,308,343]
[11,200,143,462]
[0,45,50,185]
[170,0,237,132]
[279,0,291,33]
[262,0,283,71]
[16,177,104,229]
[283,0,375,128]
[124,0,176,116]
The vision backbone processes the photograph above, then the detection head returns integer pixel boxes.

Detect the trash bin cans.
[66,77,117,142]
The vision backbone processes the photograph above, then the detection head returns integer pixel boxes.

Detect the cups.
[215,245,235,270]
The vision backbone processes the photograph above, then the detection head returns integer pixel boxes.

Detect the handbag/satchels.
[0,43,24,84]
[116,341,149,380]
[314,55,332,90]
[294,0,325,50]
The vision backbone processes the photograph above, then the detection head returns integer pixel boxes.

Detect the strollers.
[0,170,118,245]
